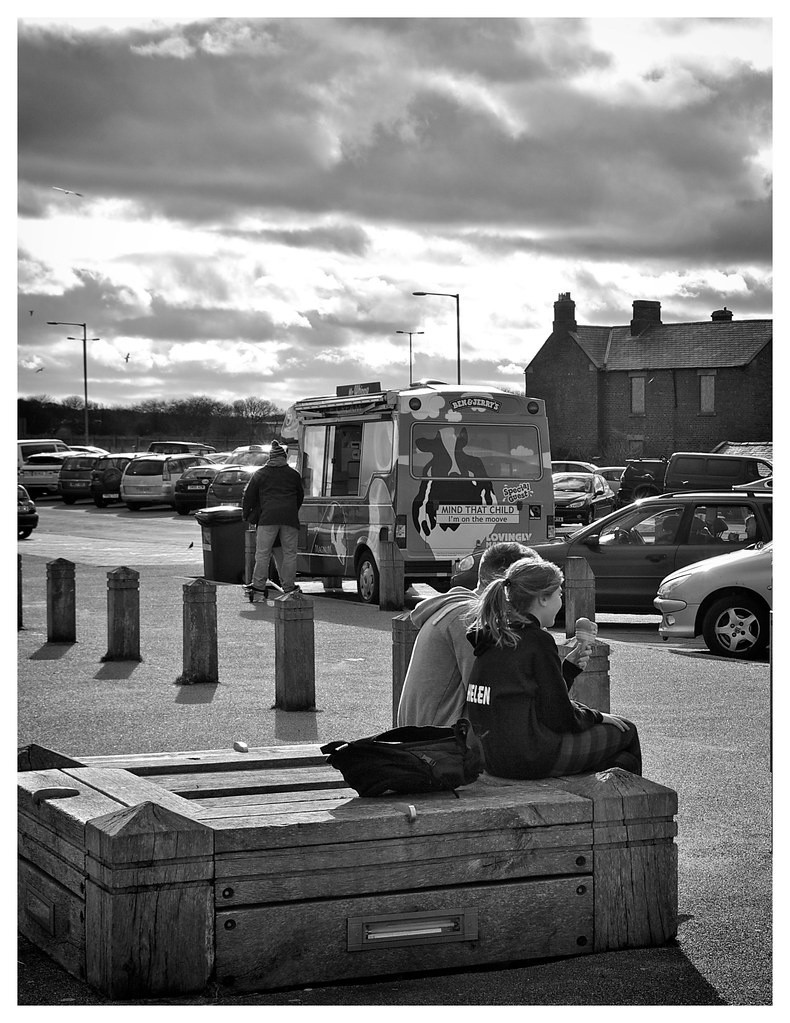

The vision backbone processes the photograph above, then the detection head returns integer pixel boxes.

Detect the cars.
[654,539,772,661]
[205,465,264,511]
[204,451,231,464]
[68,444,112,455]
[17,484,39,542]
[550,472,617,527]
[20,451,90,498]
[590,466,627,496]
[58,452,113,507]
[145,443,297,469]
[172,464,245,515]
[731,476,774,495]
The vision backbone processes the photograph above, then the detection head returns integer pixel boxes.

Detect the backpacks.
[320,718,483,799]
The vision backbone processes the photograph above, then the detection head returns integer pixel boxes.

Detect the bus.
[273,378,556,604]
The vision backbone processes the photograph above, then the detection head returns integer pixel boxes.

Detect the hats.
[269,440,287,459]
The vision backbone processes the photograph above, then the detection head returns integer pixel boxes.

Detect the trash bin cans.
[195,505,247,584]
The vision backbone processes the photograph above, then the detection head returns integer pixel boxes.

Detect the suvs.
[94,451,166,511]
[616,456,672,509]
[119,452,215,510]
[447,484,772,629]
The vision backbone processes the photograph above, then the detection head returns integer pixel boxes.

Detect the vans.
[18,439,72,473]
[551,459,599,474]
[664,451,773,492]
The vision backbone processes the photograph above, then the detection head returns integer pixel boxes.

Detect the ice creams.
[575,617,591,653]
[590,622,598,645]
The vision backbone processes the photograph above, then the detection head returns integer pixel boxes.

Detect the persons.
[655,516,679,544]
[397,541,543,729]
[728,515,757,543]
[242,441,304,593]
[465,558,643,778]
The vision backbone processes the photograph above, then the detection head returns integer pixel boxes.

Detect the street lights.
[396,330,425,387]
[46,321,89,444]
[412,291,462,386]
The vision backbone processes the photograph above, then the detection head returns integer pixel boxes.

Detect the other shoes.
[285,585,300,594]
[242,583,264,594]
